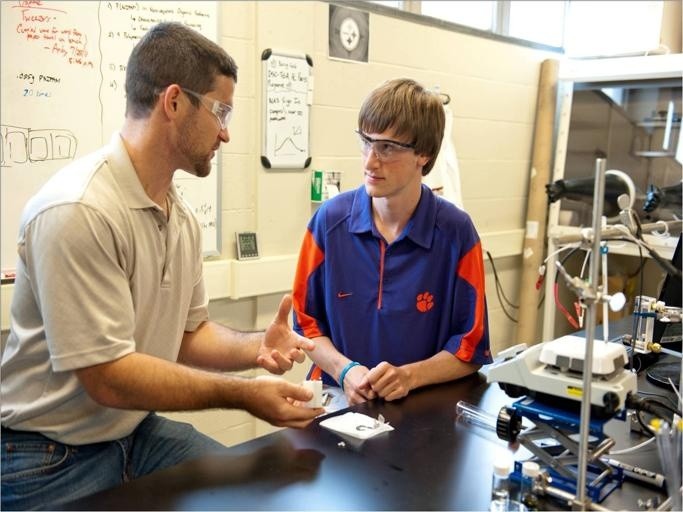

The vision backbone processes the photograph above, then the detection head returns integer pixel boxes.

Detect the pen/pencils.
[321,392,329,406]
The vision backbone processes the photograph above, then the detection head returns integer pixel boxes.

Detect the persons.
[291,78,494,423]
[1,22,325,511]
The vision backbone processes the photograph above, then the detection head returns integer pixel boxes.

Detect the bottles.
[490,461,541,511]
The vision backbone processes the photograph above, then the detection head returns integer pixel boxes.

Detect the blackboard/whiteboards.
[0,1,222,283]
[261,49,314,170]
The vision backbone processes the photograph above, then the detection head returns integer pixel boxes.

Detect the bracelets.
[339,361,359,392]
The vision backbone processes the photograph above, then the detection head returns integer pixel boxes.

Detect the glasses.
[354,130,416,164]
[155,86,232,130]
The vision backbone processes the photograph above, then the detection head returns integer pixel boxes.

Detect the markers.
[602,458,667,490]
